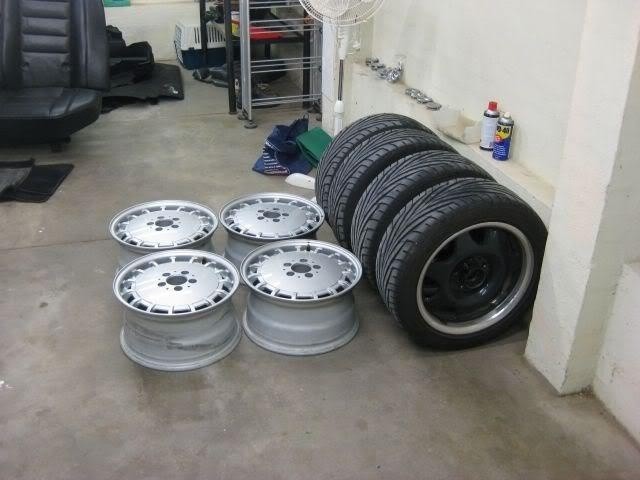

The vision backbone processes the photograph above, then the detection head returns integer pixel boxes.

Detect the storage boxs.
[175,22,227,69]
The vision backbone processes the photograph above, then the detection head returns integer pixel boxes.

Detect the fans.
[286,0,384,202]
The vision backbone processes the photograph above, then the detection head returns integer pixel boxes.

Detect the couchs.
[0,0,108,153]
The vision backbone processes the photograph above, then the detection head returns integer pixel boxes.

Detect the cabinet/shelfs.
[200,0,312,113]
